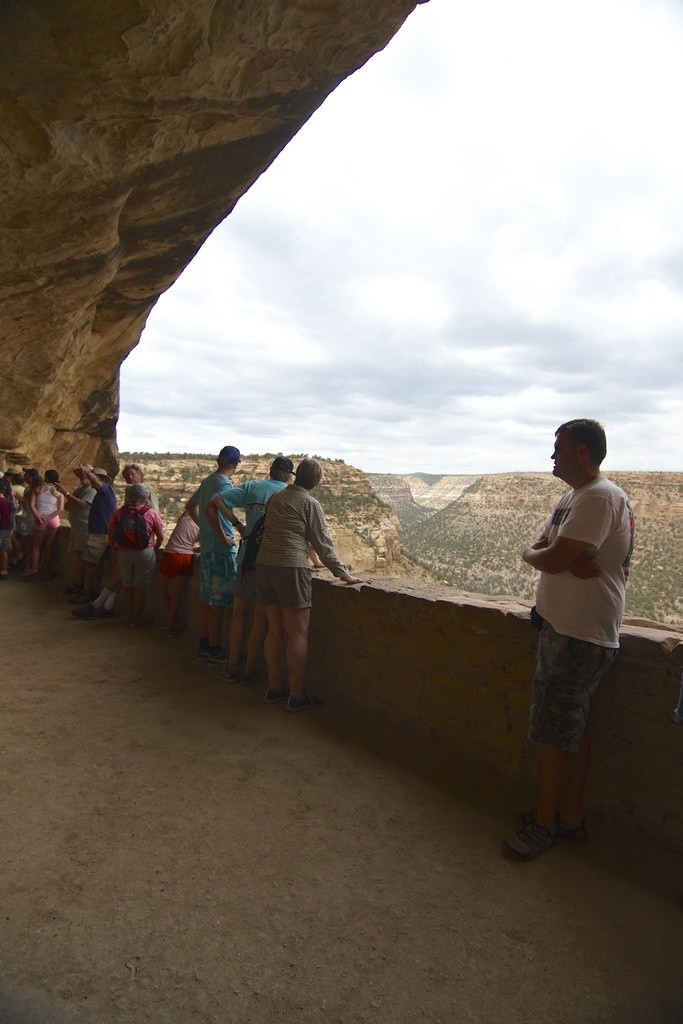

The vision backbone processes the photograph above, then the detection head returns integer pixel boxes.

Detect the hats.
[91,468,112,482]
[218,446,242,464]
[131,484,151,501]
[272,456,297,475]
[73,464,93,476]
[6,467,21,475]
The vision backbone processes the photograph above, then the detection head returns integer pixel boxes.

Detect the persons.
[502,420,634,860]
[158,510,201,639]
[71,464,161,619]
[215,456,327,686]
[108,484,165,628]
[184,445,241,663]
[0,464,117,605]
[253,459,375,712]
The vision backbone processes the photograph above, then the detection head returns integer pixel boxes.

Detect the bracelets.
[232,520,239,527]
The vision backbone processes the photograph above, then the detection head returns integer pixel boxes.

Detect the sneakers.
[500,821,563,861]
[518,809,587,844]
[286,690,324,712]
[265,684,286,701]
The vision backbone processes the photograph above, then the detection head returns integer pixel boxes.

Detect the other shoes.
[63,581,84,592]
[199,644,210,655]
[222,668,242,682]
[207,648,245,665]
[66,591,90,603]
[241,671,265,684]
[130,617,143,628]
[85,608,116,619]
[91,590,98,600]
[72,605,100,618]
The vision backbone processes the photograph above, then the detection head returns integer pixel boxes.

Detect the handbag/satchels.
[241,514,266,572]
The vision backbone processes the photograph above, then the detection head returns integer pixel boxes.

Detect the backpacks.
[117,504,151,550]
